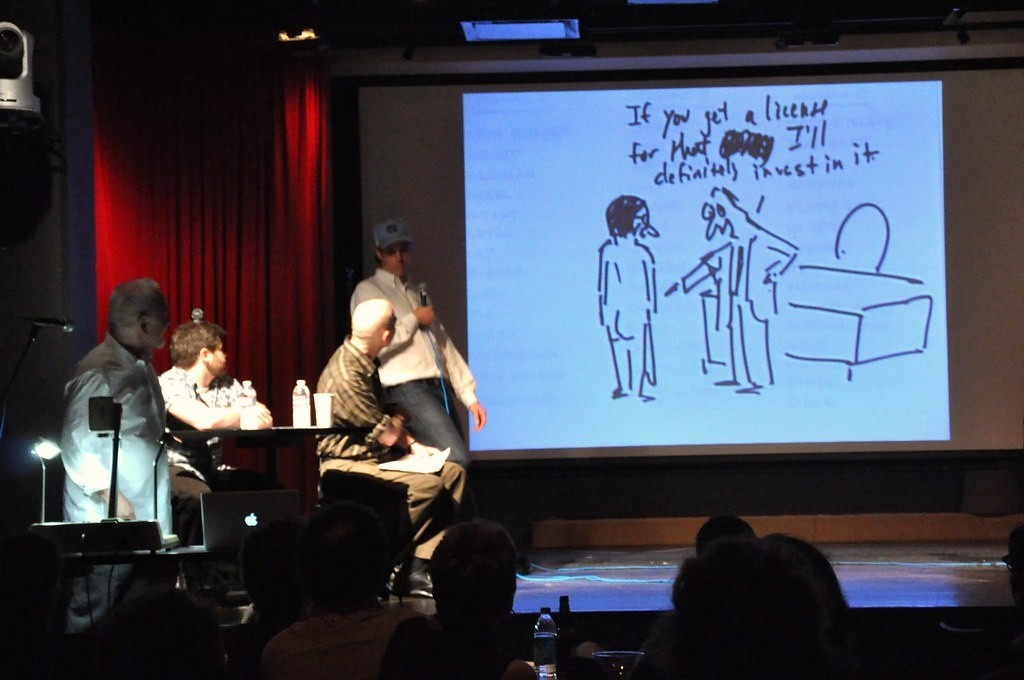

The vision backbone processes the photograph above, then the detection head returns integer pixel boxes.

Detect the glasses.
[1000,555,1024,572]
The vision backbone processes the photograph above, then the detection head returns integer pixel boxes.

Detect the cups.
[313,393,335,428]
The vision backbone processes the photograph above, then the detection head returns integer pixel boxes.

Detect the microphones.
[419,282,429,331]
[18,316,75,333]
[191,308,204,323]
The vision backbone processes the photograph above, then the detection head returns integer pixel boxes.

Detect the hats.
[375,219,414,249]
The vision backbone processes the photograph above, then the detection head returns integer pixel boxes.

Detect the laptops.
[189,489,301,552]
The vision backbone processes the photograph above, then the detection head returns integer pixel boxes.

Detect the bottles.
[292,380,311,429]
[240,380,257,431]
[533,607,557,680]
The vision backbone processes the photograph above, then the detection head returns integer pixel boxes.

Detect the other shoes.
[406,565,436,597]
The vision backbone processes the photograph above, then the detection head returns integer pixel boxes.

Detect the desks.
[67,546,217,582]
[166,427,372,522]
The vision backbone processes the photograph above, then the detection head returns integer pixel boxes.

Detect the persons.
[350,219,487,468]
[259,501,426,680]
[669,534,853,680]
[983,527,1024,680]
[59,278,172,633]
[225,513,314,680]
[157,322,273,544]
[0,529,66,680]
[634,514,756,680]
[317,299,466,599]
[377,518,538,680]
[94,586,225,680]
